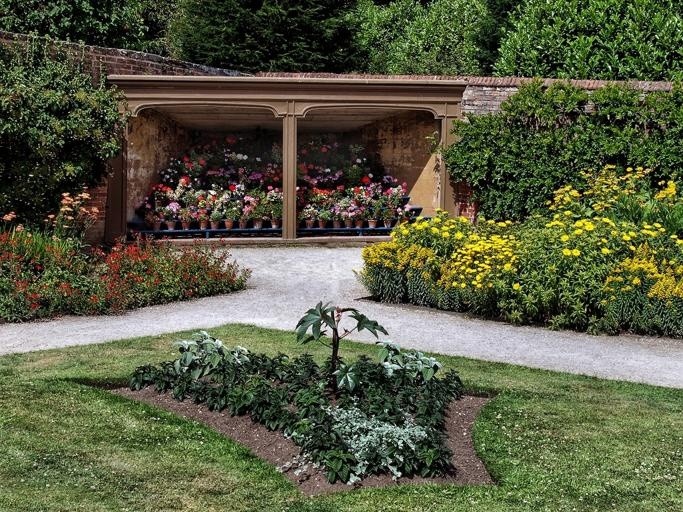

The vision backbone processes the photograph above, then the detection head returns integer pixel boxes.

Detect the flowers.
[140,134,424,225]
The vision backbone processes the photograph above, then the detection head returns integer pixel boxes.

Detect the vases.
[149,218,394,230]
